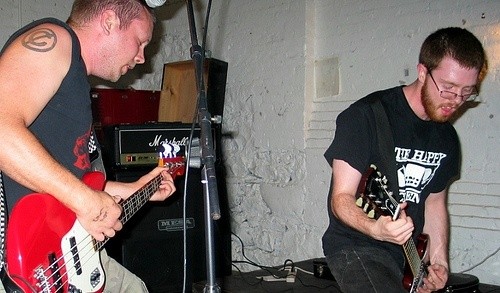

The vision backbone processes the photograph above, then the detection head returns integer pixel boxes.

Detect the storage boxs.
[88,89,159,127]
[158,57,228,127]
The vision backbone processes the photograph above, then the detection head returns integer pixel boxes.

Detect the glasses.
[425,65,478,102]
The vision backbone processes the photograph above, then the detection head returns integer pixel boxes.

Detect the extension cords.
[286,269,298,283]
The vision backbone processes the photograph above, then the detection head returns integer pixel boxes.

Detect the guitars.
[355,164,432,293]
[5,152,188,293]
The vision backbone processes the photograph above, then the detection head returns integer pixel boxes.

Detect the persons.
[322,27,483,293]
[0,0,176,292]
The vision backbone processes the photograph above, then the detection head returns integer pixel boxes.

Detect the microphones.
[145,0,166,8]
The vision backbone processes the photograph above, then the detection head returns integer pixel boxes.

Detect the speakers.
[103,167,232,293]
